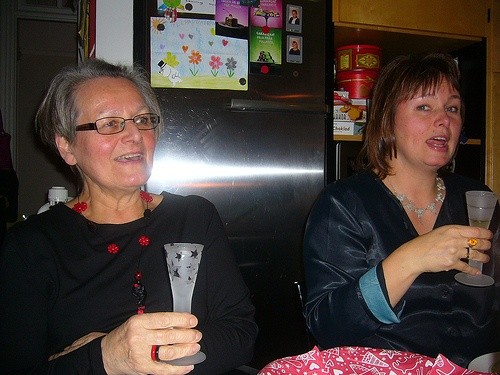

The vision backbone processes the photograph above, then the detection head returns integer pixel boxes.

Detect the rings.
[151,345,161,361]
[467,238,478,248]
[465,247,469,259]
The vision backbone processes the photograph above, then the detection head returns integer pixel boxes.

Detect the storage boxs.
[333,91,369,135]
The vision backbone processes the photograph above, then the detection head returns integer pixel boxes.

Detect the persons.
[289,10,299,25]
[289,41,300,55]
[302,44,500,374]
[0,57,259,375]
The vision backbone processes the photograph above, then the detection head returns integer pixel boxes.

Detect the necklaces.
[72,189,153,315]
[396,173,446,218]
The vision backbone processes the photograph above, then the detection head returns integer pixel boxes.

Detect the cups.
[455,192,497,286]
[161,244,207,366]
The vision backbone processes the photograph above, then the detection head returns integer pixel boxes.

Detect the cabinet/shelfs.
[333,0,500,196]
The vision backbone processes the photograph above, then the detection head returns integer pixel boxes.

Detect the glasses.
[76,113,161,135]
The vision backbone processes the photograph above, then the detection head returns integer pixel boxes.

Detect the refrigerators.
[132,0,326,375]
